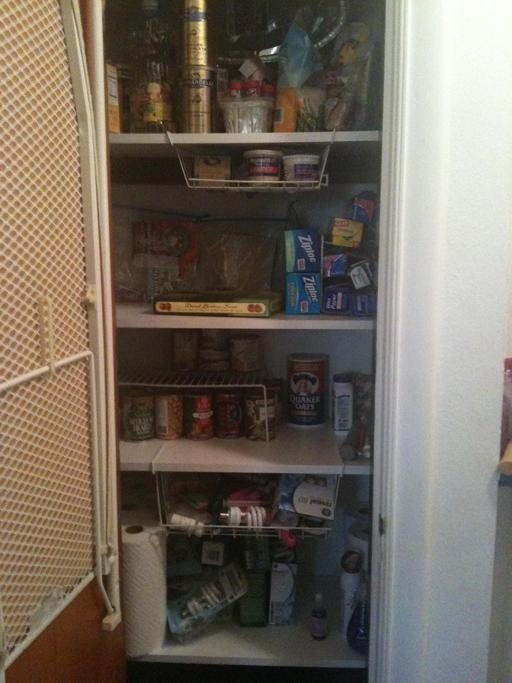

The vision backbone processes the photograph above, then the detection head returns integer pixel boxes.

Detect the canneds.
[244,148,320,186]
[120,328,329,440]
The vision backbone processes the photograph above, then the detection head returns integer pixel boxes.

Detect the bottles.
[129,1,177,133]
[347,582,370,651]
[310,594,327,644]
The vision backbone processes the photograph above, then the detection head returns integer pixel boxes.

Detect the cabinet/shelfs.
[75,0,411,666]
